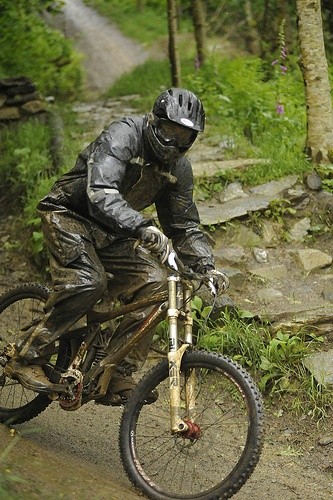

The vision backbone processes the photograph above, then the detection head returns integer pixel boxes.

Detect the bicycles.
[0,230,268,500]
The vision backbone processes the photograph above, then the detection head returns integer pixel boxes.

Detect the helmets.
[149,87,205,165]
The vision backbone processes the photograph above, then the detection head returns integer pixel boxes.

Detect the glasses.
[154,118,197,151]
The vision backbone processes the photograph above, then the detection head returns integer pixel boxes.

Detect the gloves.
[135,223,171,265]
[198,264,230,297]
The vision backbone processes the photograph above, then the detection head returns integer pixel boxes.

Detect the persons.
[0,87,219,407]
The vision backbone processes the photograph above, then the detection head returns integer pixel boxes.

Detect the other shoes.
[4,354,54,394]
[109,370,159,405]
